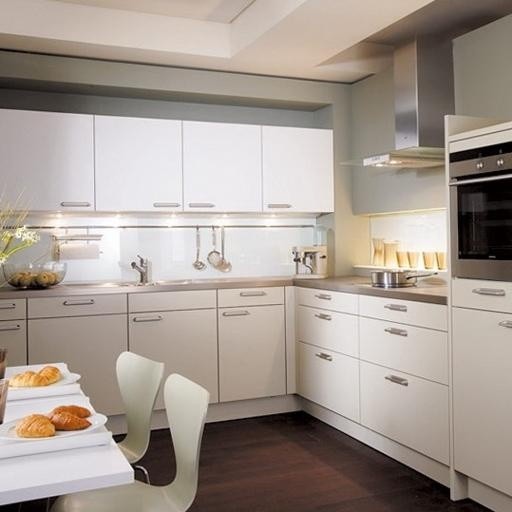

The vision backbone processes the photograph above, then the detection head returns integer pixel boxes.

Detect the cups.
[395,250,447,270]
[369,236,400,267]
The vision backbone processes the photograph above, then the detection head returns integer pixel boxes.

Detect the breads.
[15,405,91,438]
[9,365,59,387]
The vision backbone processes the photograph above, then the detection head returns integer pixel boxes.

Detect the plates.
[13,371,81,387]
[0,410,110,442]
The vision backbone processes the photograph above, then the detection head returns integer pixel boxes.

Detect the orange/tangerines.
[8,271,60,290]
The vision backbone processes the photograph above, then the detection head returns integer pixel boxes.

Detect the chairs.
[113,352,167,485]
[49,370,211,512]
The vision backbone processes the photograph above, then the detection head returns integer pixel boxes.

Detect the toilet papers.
[51,243,100,261]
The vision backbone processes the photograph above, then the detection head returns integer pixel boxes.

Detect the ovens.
[449,140,512,282]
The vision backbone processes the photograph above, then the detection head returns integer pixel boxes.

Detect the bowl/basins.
[1,262,68,289]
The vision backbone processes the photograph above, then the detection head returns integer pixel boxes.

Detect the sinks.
[58,282,131,288]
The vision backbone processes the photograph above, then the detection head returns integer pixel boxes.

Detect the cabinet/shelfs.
[1,285,302,438]
[295,286,511,512]
[94,114,262,213]
[0,108,95,213]
[262,125,334,213]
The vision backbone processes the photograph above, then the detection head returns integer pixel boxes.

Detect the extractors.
[338,34,455,172]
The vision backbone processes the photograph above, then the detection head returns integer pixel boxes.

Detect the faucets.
[130,254,149,284]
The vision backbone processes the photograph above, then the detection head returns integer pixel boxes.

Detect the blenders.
[291,225,329,281]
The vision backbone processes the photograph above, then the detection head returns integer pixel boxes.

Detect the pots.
[370,270,438,288]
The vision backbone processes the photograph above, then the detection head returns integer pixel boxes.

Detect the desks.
[1,362,136,509]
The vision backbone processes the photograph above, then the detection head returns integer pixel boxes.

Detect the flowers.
[0,190,41,285]
